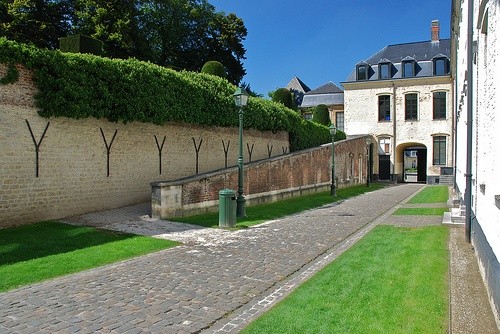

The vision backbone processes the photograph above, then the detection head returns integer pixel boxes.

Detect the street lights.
[231,86,249,216]
[327,124,337,197]
[366,138,372,187]
